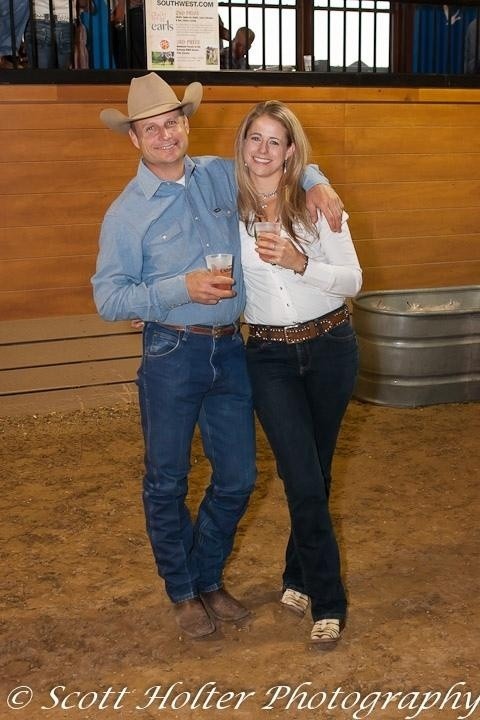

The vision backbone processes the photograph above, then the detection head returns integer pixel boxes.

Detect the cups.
[304,55,313,72]
[254,221,283,261]
[205,256,236,300]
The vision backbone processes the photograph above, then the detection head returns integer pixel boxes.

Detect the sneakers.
[197,585,253,625]
[173,595,217,641]
[280,584,312,616]
[307,612,347,646]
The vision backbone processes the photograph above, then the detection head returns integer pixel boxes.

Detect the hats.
[98,71,204,135]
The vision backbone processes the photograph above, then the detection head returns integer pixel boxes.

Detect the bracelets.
[292,252,310,275]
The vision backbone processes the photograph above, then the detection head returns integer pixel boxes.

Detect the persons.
[2,0,258,73]
[130,100,363,644]
[90,72,346,635]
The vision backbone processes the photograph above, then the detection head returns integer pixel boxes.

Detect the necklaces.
[256,185,279,210]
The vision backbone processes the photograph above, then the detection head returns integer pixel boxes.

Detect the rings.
[271,242,277,251]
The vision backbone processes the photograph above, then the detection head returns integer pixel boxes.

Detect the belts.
[151,315,242,338]
[246,303,350,345]
